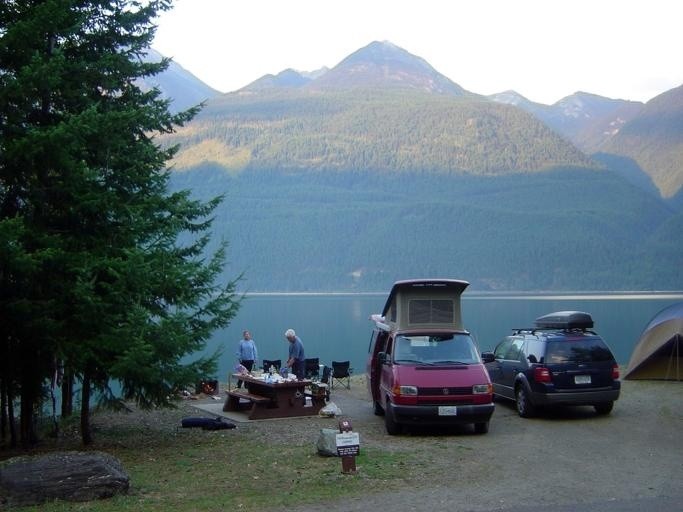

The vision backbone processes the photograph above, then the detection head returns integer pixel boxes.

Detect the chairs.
[332,361,351,389]
[398,338,417,360]
[263,360,281,373]
[305,358,319,380]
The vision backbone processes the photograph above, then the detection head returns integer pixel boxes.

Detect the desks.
[231,374,311,420]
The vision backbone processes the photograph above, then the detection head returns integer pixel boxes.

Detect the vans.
[366,276,495,435]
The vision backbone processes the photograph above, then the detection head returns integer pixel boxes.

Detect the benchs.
[223,390,328,420]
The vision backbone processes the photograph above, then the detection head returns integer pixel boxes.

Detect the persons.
[285,329,306,405]
[236,330,257,388]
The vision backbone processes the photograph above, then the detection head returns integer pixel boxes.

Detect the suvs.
[482,309,621,417]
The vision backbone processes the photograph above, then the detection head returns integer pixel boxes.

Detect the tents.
[623,301,683,380]
